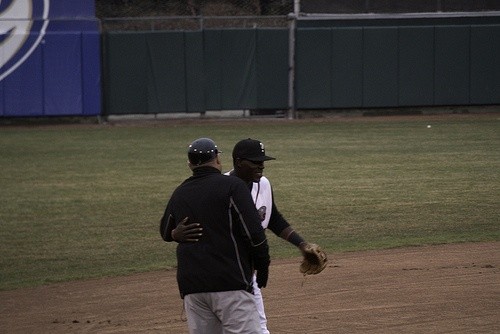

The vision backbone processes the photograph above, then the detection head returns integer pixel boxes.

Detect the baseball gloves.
[299,243,327,275]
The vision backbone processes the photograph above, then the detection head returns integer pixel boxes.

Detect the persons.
[159,137,271,334]
[171,138,328,334]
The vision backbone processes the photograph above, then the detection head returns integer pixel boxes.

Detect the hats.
[187,138,223,165]
[232,138,276,162]
